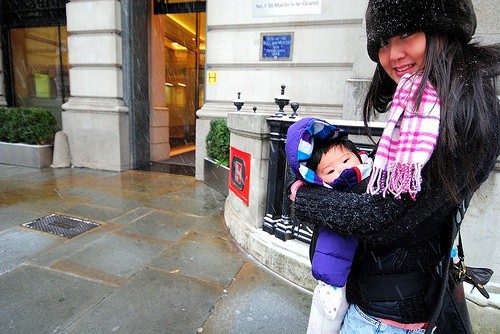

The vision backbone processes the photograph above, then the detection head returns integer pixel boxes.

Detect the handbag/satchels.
[433,257,493,334]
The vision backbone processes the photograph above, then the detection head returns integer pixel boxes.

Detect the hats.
[366,0,477,63]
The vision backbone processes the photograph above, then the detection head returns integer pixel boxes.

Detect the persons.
[284,117,374,334]
[286,0,500,334]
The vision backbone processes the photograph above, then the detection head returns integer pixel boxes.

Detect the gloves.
[321,283,342,321]
